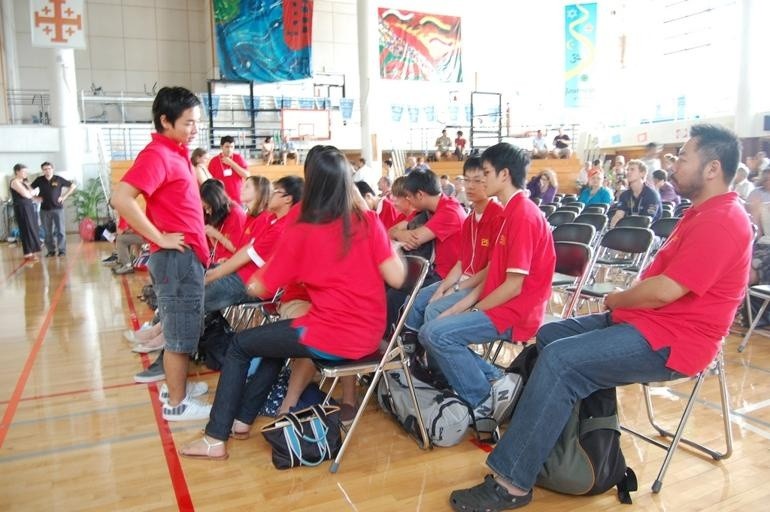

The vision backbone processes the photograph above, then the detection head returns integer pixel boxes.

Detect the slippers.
[449,473,532,512]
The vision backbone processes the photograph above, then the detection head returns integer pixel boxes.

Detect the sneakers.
[47,251,55,256]
[24,256,40,263]
[59,252,65,257]
[124,322,211,420]
[473,372,524,428]
[103,254,134,275]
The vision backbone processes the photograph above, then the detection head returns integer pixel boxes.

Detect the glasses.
[460,179,483,186]
[271,188,289,196]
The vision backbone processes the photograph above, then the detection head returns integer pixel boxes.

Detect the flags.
[30,1,86,53]
[213,1,314,84]
[377,7,464,84]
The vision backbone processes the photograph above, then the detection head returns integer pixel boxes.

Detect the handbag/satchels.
[401,209,435,266]
[95,221,116,241]
[79,217,98,241]
[259,405,340,470]
[195,312,236,370]
[377,368,470,447]
[132,244,151,270]
[259,368,289,417]
[505,344,625,496]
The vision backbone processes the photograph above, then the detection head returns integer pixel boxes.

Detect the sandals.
[178,436,229,460]
[200,419,251,441]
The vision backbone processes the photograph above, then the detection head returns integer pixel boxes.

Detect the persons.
[29,161,78,258]
[101,87,769,511]
[9,162,44,261]
